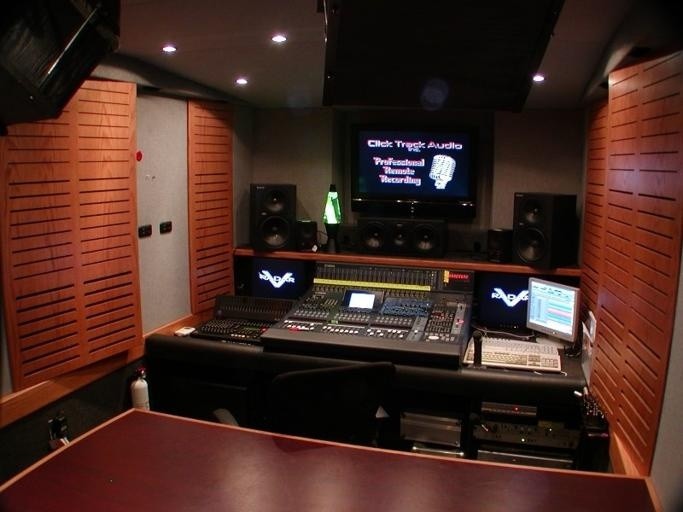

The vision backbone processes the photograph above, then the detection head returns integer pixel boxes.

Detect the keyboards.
[462,336,562,373]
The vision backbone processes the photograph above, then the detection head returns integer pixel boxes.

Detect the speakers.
[347,218,447,260]
[512,191,578,272]
[1,2,121,121]
[323,0,565,114]
[297,220,318,251]
[486,228,511,263]
[249,183,298,252]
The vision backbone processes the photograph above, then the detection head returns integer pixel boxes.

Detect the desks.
[0,408,662,512]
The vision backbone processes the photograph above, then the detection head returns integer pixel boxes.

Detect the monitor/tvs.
[233,257,313,299]
[349,121,480,220]
[527,277,582,349]
[342,289,384,309]
[471,271,530,336]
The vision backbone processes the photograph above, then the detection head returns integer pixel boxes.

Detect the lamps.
[322,184,344,255]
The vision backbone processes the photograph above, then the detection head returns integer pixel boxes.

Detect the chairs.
[270,361,408,453]
